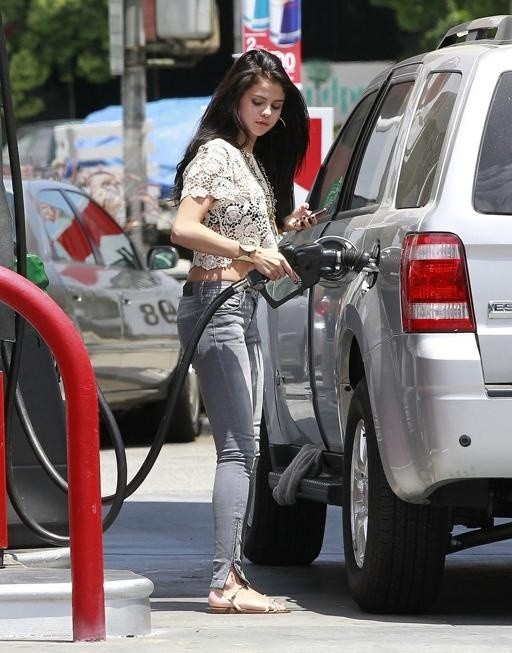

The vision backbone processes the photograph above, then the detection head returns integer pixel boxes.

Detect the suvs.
[239,14,512,613]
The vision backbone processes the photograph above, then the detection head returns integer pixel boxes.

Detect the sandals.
[205,583,291,614]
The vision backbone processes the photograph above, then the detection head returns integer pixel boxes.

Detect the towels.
[272,444,325,506]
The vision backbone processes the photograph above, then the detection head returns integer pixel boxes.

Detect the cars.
[5,180,202,444]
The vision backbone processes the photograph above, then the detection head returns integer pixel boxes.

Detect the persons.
[168,48,328,616]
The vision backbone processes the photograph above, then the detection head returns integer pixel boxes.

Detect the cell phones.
[291,205,331,229]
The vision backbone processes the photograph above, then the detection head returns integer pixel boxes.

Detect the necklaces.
[234,141,279,237]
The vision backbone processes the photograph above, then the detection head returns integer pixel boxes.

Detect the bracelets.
[232,241,258,264]
